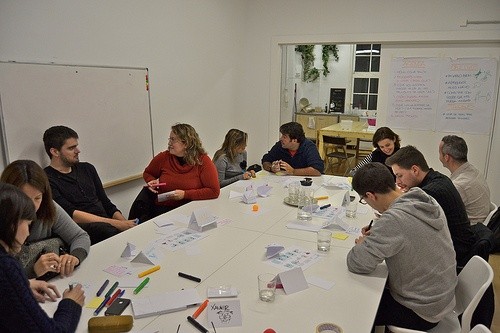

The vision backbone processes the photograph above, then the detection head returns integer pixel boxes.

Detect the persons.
[212,129,256,188]
[386,146,472,274]
[43,126,137,246]
[143,124,220,200]
[439,135,490,225]
[350,127,401,176]
[262,122,324,175]
[347,163,458,333]
[0,182,85,333]
[0,160,91,282]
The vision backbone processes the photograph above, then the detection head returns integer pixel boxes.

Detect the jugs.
[297,187,312,221]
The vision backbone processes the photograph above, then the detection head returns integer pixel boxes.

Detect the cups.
[257,273,277,302]
[345,202,358,218]
[288,183,313,205]
[316,231,332,252]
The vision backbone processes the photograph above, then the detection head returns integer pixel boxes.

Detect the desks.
[319,121,376,174]
[36,163,403,333]
[295,114,359,146]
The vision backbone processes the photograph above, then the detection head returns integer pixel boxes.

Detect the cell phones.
[37,271,60,282]
[104,298,131,317]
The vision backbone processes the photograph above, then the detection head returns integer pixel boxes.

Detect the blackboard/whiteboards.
[0,61,155,190]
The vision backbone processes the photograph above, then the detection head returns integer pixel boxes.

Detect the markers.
[316,196,328,200]
[138,266,160,278]
[94,289,125,316]
[192,299,209,318]
[97,279,109,296]
[321,204,331,210]
[242,171,251,178]
[134,277,150,294]
[187,316,209,333]
[367,219,373,231]
[178,272,201,282]
[69,284,73,291]
[145,183,166,188]
[105,282,119,297]
[267,284,283,289]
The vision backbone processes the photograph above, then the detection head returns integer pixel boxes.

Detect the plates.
[284,196,317,206]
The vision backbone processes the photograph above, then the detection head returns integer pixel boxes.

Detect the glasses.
[358,191,368,204]
[168,137,185,143]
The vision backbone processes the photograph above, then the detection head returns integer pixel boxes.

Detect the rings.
[50,265,55,268]
[66,261,71,264]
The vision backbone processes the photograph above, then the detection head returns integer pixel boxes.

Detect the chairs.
[322,136,355,179]
[375,200,499,333]
